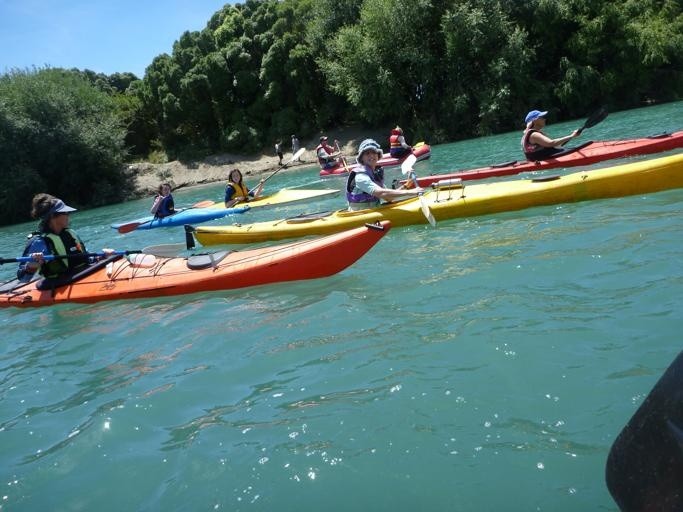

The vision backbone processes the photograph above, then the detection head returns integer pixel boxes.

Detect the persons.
[343,138,425,211]
[388,125,412,159]
[274,137,284,167]
[222,168,265,211]
[290,134,301,161]
[148,183,180,218]
[518,110,578,160]
[17,194,112,284]
[315,136,347,171]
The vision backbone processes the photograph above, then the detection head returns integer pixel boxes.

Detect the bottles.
[126,253,157,268]
[431,178,464,191]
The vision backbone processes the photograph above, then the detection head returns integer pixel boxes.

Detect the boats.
[400,131,683,191]
[319,141,431,179]
[1,219,395,307]
[110,205,252,235]
[188,188,341,209]
[182,152,683,251]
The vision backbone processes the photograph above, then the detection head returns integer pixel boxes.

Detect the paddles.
[230,147,306,208]
[401,154,437,227]
[0,242,185,265]
[118,200,214,233]
[410,141,425,151]
[335,141,350,174]
[561,105,608,147]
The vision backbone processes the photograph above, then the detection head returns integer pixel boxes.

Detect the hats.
[42,200,77,220]
[525,110,549,122]
[320,136,327,141]
[356,139,383,165]
